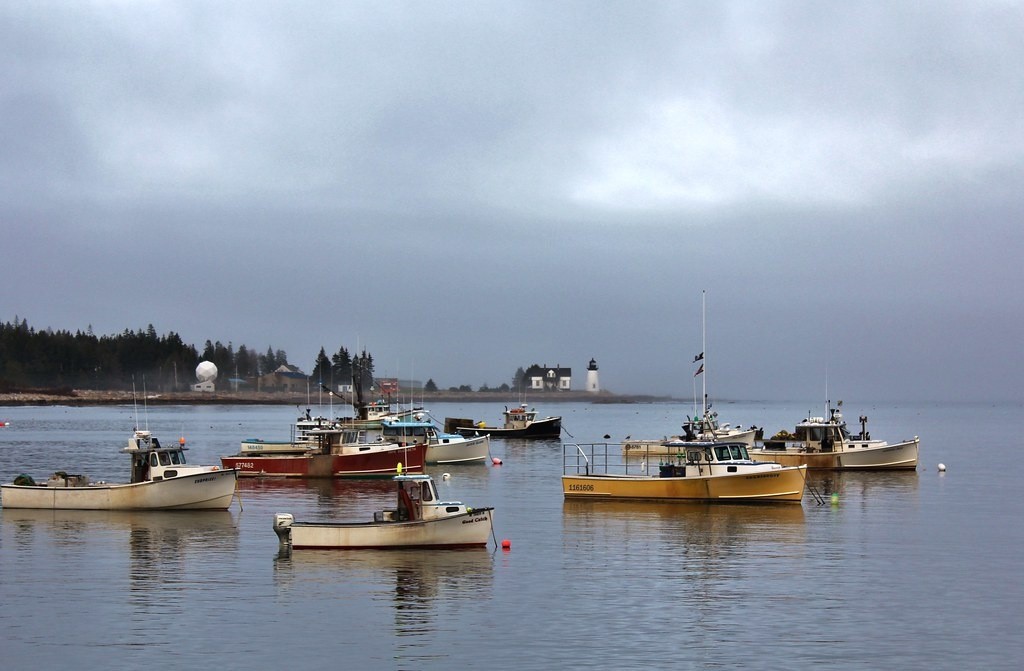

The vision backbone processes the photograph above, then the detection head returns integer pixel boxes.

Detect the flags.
[695,364,704,376]
[695,352,704,361]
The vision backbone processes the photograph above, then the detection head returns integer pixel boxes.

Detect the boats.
[749,371,920,470]
[442,385,562,439]
[330,421,490,464]
[1,374,241,510]
[240,357,431,456]
[273,462,494,557]
[562,440,809,505]
[219,430,428,478]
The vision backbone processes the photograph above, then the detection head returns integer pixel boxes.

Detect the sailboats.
[620,288,758,456]
